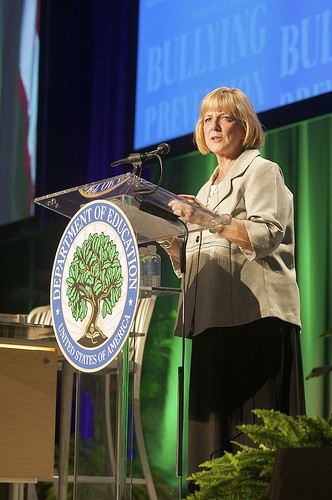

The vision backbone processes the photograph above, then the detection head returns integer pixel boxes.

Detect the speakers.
[267,447,331,500]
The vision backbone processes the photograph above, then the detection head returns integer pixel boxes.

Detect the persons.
[150,85,305,496]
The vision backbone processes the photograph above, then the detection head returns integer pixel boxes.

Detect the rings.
[179,209,185,217]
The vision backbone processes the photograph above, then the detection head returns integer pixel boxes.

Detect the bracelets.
[209,212,221,234]
[156,237,177,249]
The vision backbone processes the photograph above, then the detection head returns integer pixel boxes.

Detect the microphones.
[111,143,170,166]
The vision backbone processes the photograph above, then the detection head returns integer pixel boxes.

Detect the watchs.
[216,213,233,234]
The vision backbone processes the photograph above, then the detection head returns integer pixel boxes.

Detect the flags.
[14,0,36,215]
